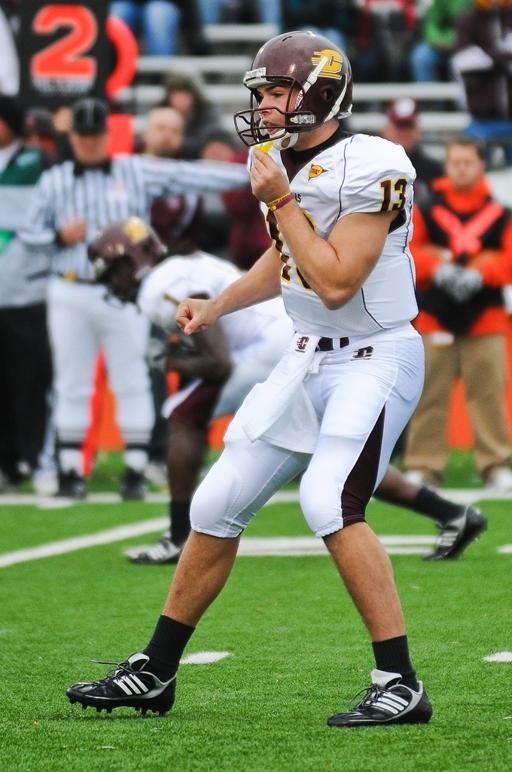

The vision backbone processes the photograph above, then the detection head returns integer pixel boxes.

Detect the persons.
[83,214,491,568]
[0,0,511,500]
[61,26,434,729]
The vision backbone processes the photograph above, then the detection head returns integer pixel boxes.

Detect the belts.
[314,338,350,351]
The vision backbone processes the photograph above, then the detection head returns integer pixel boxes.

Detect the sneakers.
[486,466,512,495]
[118,467,146,503]
[124,533,184,563]
[404,469,435,492]
[66,654,178,717]
[420,502,486,560]
[54,469,88,500]
[326,669,432,726]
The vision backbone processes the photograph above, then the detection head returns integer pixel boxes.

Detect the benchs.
[120,50,474,138]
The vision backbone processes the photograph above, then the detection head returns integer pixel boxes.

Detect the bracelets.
[269,194,296,212]
[264,190,290,205]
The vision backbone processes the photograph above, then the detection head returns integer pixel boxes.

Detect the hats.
[70,99,110,136]
[388,97,419,126]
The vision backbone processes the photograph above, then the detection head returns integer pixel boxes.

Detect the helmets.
[233,30,354,152]
[88,193,202,303]
